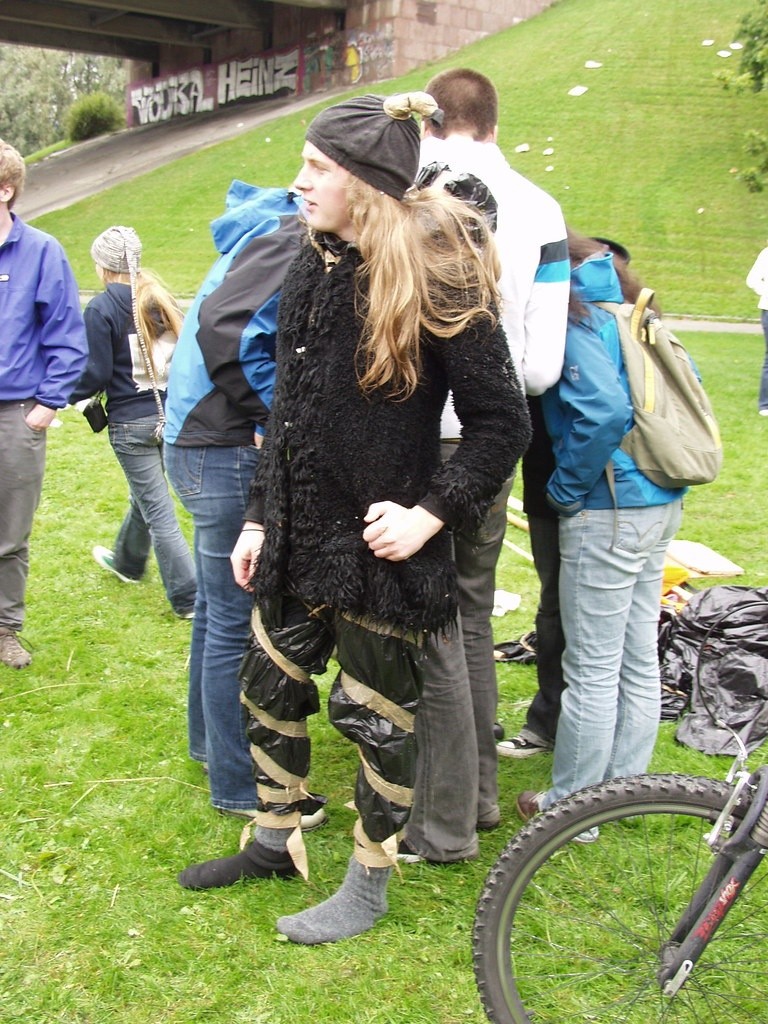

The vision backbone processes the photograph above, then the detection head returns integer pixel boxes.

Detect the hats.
[306,92,445,200]
[90,225,167,443]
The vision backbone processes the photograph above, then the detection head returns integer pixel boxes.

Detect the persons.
[175,92,533,945]
[0,140,88,667]
[745,247,768,416]
[67,226,197,621]
[162,67,695,867]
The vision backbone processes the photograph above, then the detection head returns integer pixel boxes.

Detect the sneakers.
[1,626,35,669]
[495,735,550,758]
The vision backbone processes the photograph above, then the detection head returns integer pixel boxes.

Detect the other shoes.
[218,797,330,832]
[174,607,197,619]
[92,546,142,585]
[397,834,480,863]
[516,791,600,843]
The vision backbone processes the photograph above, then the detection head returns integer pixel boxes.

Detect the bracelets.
[241,528,264,532]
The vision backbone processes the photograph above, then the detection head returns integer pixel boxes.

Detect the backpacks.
[586,286,723,490]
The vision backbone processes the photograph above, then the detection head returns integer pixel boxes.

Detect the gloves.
[546,491,587,518]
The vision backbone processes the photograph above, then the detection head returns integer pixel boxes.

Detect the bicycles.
[469,602,768,1024]
